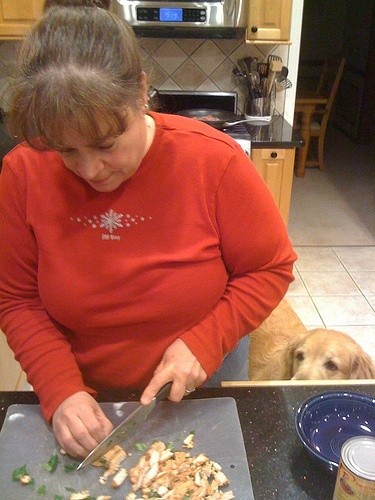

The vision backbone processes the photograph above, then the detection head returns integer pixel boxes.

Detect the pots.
[177,109,273,129]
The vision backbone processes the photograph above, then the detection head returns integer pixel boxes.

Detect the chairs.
[292,55,346,169]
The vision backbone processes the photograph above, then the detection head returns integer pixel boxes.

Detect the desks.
[293,85,328,177]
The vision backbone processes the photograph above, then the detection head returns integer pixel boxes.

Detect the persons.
[0,0,298,460]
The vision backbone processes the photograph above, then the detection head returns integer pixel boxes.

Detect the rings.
[185,388,195,393]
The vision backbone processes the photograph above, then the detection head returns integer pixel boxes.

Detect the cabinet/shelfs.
[0,0,43,42]
[250,148,297,226]
[243,0,292,45]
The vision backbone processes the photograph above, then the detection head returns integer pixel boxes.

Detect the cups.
[246,97,271,116]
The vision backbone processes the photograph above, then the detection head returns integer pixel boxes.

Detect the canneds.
[333,436,375,500]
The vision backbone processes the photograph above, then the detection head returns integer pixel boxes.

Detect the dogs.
[249,295,375,374]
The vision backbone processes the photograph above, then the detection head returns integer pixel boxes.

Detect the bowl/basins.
[292,390,375,474]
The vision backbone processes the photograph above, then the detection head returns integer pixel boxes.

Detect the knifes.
[77,383,173,469]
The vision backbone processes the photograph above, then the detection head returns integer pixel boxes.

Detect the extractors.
[109,0,246,40]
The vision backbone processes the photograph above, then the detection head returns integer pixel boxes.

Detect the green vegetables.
[12,421,218,500]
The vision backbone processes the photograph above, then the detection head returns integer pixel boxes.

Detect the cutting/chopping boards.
[0,396,255,500]
